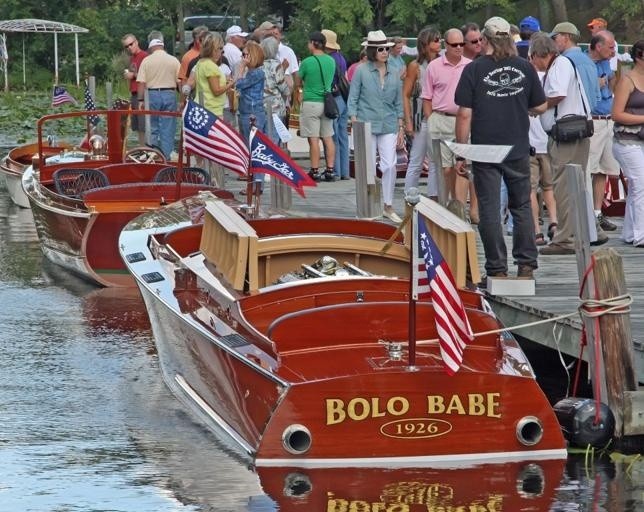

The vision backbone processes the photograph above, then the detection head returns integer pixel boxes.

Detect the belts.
[545,130,553,137]
[433,109,457,116]
[148,87,175,91]
[129,91,137,96]
[591,114,613,120]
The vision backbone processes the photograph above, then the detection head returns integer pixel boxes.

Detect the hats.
[148,39,165,48]
[309,31,325,41]
[361,30,396,47]
[588,17,608,25]
[485,16,512,38]
[225,25,249,37]
[321,29,341,50]
[548,22,582,37]
[520,15,541,32]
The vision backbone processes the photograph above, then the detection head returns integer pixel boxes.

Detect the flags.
[181,98,250,176]
[249,125,318,198]
[83,80,101,126]
[411,208,475,377]
[52,84,78,107]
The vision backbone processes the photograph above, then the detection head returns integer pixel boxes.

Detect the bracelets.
[399,125,404,128]
[456,156,465,161]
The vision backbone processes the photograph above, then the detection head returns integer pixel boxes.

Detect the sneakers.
[321,171,339,182]
[239,188,263,196]
[306,171,321,182]
[596,215,618,231]
[383,210,404,224]
[588,222,608,246]
[516,265,535,280]
[539,243,575,255]
[476,272,507,288]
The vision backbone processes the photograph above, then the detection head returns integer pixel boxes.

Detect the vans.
[176,15,256,54]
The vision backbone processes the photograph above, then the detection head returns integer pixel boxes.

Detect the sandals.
[548,223,558,241]
[534,233,546,245]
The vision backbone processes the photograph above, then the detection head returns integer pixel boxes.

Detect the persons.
[186,30,232,80]
[522,71,558,245]
[183,52,235,127]
[347,50,370,83]
[294,32,336,183]
[223,24,250,82]
[582,30,620,231]
[256,20,274,44]
[235,40,269,196]
[421,28,473,205]
[461,23,481,59]
[528,32,593,255]
[136,38,181,161]
[257,36,292,155]
[194,31,236,190]
[147,31,164,53]
[346,30,404,222]
[259,33,290,73]
[613,39,644,248]
[510,23,522,42]
[321,29,350,182]
[454,15,548,289]
[527,32,609,255]
[515,16,541,58]
[402,28,442,205]
[121,33,149,147]
[250,28,261,44]
[388,36,407,81]
[177,25,208,110]
[549,21,602,112]
[264,24,303,135]
[588,17,618,73]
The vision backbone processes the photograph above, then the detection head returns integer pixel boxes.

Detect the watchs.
[296,89,301,93]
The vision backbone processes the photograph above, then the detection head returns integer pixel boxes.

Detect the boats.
[0,141,92,209]
[21,160,255,290]
[0,136,106,210]
[21,109,234,288]
[120,368,566,512]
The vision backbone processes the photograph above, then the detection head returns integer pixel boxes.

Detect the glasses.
[242,52,248,57]
[432,37,441,43]
[376,47,390,52]
[125,41,136,48]
[465,37,483,44]
[446,41,465,47]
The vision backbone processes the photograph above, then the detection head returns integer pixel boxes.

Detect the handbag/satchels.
[313,54,339,120]
[551,55,595,142]
